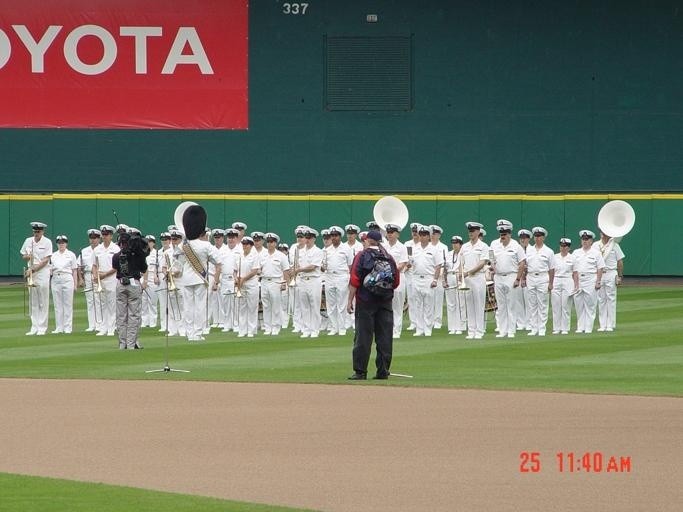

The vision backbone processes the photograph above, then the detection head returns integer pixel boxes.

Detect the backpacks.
[364,248,395,297]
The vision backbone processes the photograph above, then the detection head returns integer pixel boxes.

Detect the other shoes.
[605,328,613,331]
[392,335,399,339]
[407,326,416,330]
[449,331,455,335]
[575,329,583,333]
[37,332,45,336]
[96,332,106,336]
[26,332,36,335]
[85,328,95,331]
[65,331,71,334]
[107,333,114,336]
[466,335,474,339]
[552,331,560,335]
[413,333,422,336]
[455,330,463,335]
[597,328,605,331]
[434,325,441,328]
[425,334,431,336]
[561,330,569,335]
[473,335,482,339]
[141,325,355,339]
[495,325,545,337]
[52,330,61,334]
[584,330,592,333]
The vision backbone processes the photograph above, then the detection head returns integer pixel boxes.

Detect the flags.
[0,0,249,131]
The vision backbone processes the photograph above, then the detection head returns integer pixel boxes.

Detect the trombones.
[23,240,36,316]
[286,248,297,315]
[165,254,181,321]
[456,253,470,321]
[91,255,106,323]
[233,256,244,326]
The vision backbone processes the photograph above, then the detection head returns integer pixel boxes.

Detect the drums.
[485,281,498,312]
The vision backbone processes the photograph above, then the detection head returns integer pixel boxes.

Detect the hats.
[465,222,484,230]
[30,222,48,230]
[205,222,444,250]
[517,229,533,239]
[560,238,572,247]
[478,229,487,236]
[532,227,547,236]
[88,222,185,242]
[579,230,596,241]
[496,219,512,233]
[451,235,463,245]
[55,235,68,244]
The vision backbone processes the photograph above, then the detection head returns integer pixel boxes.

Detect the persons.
[345,231,400,380]
[18,219,626,339]
[112,236,148,349]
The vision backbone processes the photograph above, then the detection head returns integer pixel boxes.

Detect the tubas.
[373,197,409,244]
[174,202,199,249]
[598,200,635,261]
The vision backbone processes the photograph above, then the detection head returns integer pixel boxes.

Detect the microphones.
[174,252,184,256]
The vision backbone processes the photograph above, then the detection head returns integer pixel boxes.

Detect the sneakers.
[348,371,367,380]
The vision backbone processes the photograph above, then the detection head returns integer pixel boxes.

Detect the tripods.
[145,258,190,374]
